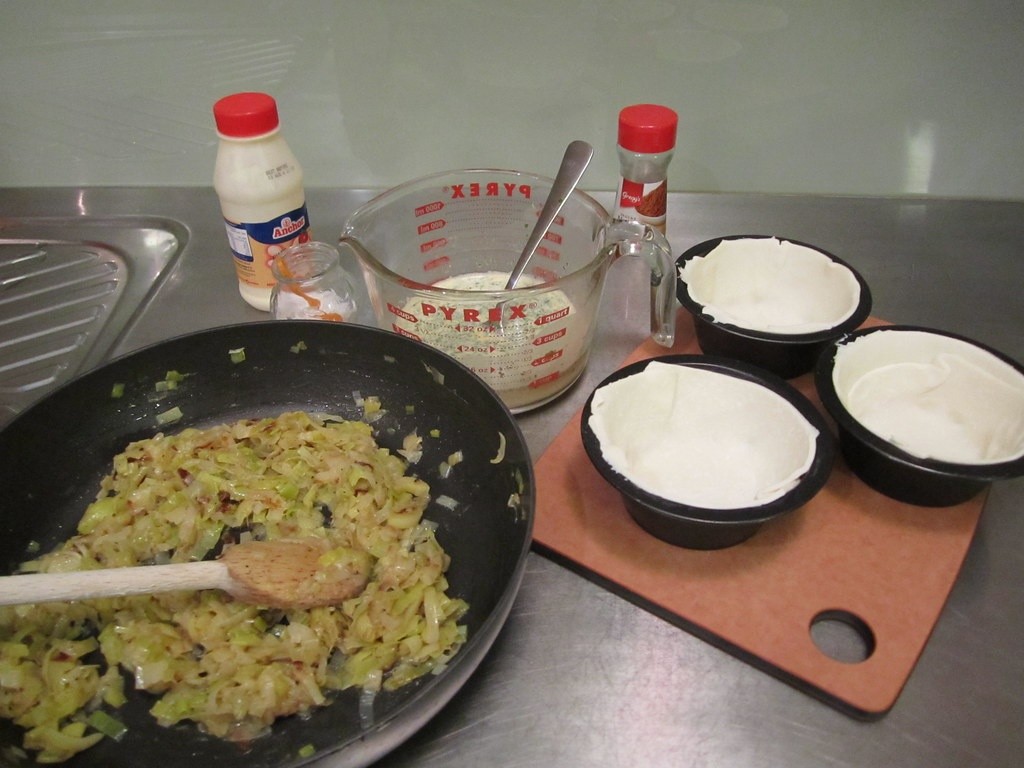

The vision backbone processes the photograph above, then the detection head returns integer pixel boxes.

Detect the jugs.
[338,163,682,417]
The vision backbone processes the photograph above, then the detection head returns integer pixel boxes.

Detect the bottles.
[607,103,682,339]
[268,241,359,325]
[210,95,312,312]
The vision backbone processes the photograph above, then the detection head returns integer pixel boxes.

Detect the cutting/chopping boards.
[529,303,993,717]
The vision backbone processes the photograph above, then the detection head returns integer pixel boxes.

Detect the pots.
[0,326,537,767]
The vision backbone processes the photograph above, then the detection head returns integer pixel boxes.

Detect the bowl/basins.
[669,234,872,380]
[577,353,834,551]
[815,329,1022,507]
